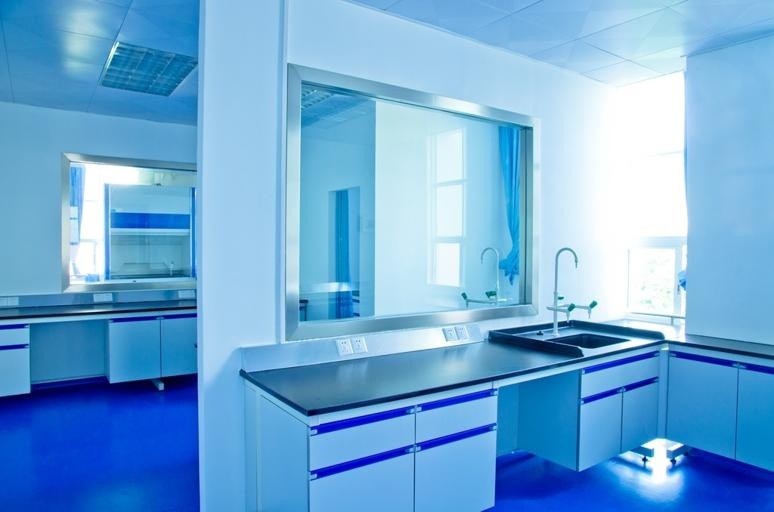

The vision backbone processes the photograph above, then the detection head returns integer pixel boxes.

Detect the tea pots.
[61,152,197,293]
[286,63,542,341]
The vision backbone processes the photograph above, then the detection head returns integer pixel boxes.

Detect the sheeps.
[480,247,500,289]
[555,248,578,292]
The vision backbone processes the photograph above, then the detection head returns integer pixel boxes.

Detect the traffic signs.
[335,338,354,357]
[350,336,368,353]
[454,325,470,340]
[442,327,458,341]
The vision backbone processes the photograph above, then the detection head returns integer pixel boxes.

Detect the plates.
[517,345,660,472]
[105,183,196,234]
[105,313,197,384]
[243,379,498,512]
[0,324,31,399]
[666,343,774,472]
